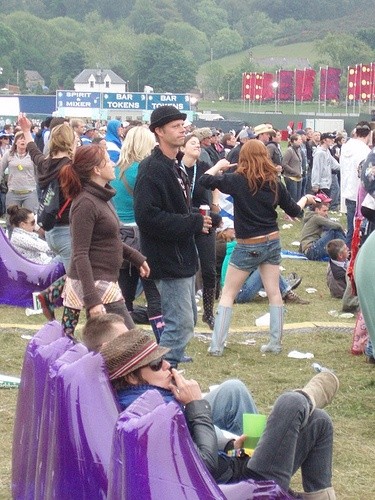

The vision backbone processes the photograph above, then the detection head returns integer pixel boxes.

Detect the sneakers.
[285,277,302,289]
[286,290,311,305]
[285,272,297,282]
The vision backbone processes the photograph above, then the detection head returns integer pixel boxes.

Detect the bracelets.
[304,195,309,204]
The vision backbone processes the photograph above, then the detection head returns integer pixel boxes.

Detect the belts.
[12,189,33,194]
[237,232,279,245]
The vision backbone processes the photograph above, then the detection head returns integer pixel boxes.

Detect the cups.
[32,292,42,310]
[242,413,267,457]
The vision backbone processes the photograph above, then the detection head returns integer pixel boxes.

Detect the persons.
[200,138,317,356]
[82,314,260,435]
[18,112,81,341]
[100,328,340,500]
[281,118,375,367]
[61,143,151,341]
[0,116,304,324]
[135,106,223,369]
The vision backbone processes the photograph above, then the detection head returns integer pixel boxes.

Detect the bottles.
[199,200,210,230]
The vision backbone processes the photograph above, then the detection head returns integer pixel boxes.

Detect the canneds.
[198,204,210,231]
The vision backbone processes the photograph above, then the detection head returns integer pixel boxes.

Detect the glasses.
[356,125,371,130]
[25,219,35,226]
[149,357,163,372]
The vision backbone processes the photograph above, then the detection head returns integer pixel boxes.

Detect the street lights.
[273,82,278,114]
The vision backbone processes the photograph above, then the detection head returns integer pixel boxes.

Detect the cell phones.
[315,198,322,203]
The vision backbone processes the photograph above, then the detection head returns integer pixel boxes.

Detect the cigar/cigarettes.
[169,370,184,376]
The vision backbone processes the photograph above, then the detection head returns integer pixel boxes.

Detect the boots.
[260,304,283,353]
[38,274,67,322]
[352,310,369,355]
[201,286,214,330]
[301,486,336,500]
[149,315,194,362]
[292,371,339,416]
[208,306,231,356]
[62,306,81,343]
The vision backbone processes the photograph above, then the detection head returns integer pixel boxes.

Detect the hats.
[101,330,172,380]
[254,124,276,135]
[193,127,212,141]
[149,105,186,132]
[316,194,331,203]
[296,129,307,135]
[0,129,13,138]
[321,133,337,139]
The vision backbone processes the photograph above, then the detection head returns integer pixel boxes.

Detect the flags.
[242,60,375,102]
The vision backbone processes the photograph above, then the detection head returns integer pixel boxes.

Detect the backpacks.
[37,182,60,231]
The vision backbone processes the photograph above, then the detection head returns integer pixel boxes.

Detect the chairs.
[0,228,63,304]
[12,319,288,500]
